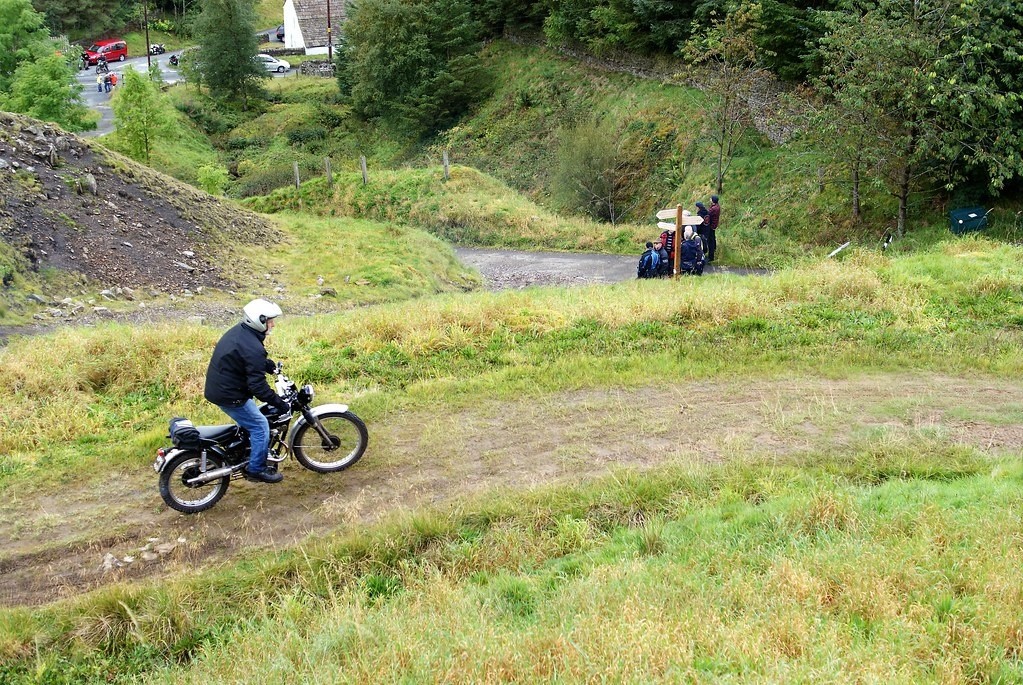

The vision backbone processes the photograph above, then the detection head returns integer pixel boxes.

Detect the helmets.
[242,298,283,333]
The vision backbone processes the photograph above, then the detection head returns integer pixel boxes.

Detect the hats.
[654,238,662,242]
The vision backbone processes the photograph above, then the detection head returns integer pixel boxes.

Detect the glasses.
[654,242,658,245]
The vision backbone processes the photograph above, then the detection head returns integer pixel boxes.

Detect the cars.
[252,54,291,73]
[276,25,285,43]
[83,39,128,65]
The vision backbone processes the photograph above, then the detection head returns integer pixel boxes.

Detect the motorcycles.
[148,361,368,513]
[96,59,108,73]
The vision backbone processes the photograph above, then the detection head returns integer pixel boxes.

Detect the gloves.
[278,402,290,413]
[266,359,276,374]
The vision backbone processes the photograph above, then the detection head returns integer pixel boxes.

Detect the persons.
[82,51,90,70]
[96,72,118,93]
[96,53,109,71]
[635,196,720,280]
[204,298,291,484]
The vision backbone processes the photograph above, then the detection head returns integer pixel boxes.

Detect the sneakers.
[248,467,283,483]
